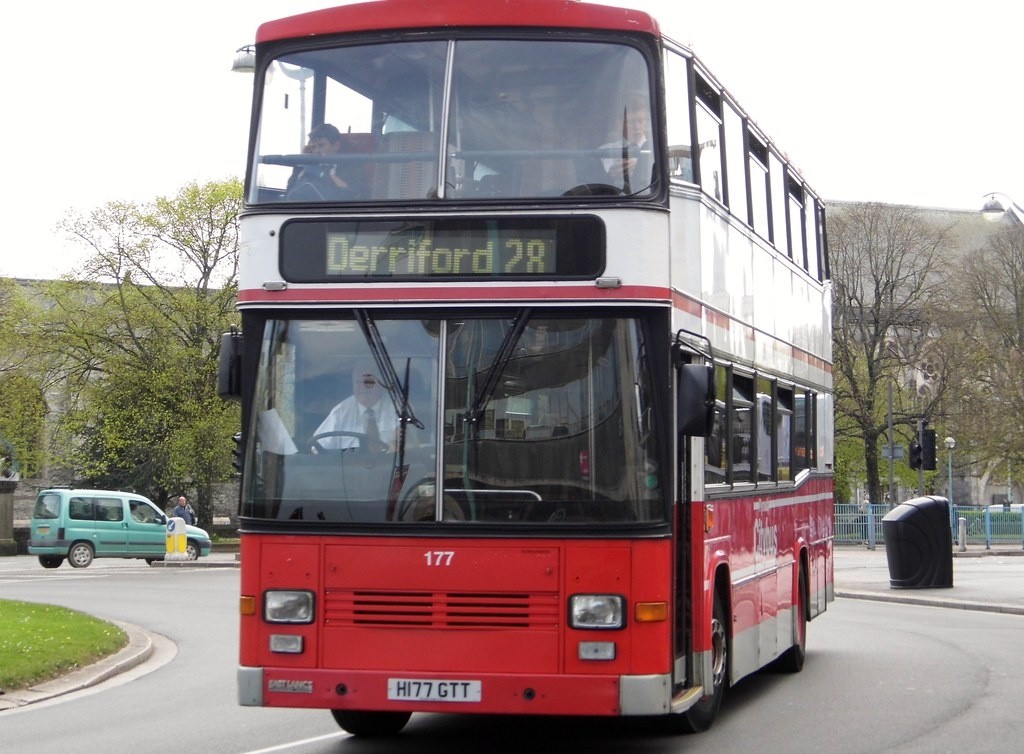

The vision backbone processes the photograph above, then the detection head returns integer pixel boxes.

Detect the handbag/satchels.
[188,505,195,524]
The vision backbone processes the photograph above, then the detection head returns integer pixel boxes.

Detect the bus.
[218,3,836,735]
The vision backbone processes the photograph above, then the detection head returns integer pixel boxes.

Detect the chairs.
[338,131,442,201]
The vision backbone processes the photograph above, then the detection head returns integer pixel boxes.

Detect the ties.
[364,408,380,453]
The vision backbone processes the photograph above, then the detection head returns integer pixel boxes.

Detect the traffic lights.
[908,442,923,469]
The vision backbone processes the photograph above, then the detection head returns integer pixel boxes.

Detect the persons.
[286,124,355,202]
[597,95,659,191]
[131,506,146,523]
[174,497,196,526]
[310,359,417,453]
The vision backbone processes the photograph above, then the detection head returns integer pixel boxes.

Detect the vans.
[27,487,212,568]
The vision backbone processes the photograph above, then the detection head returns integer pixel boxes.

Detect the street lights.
[944,435,956,527]
